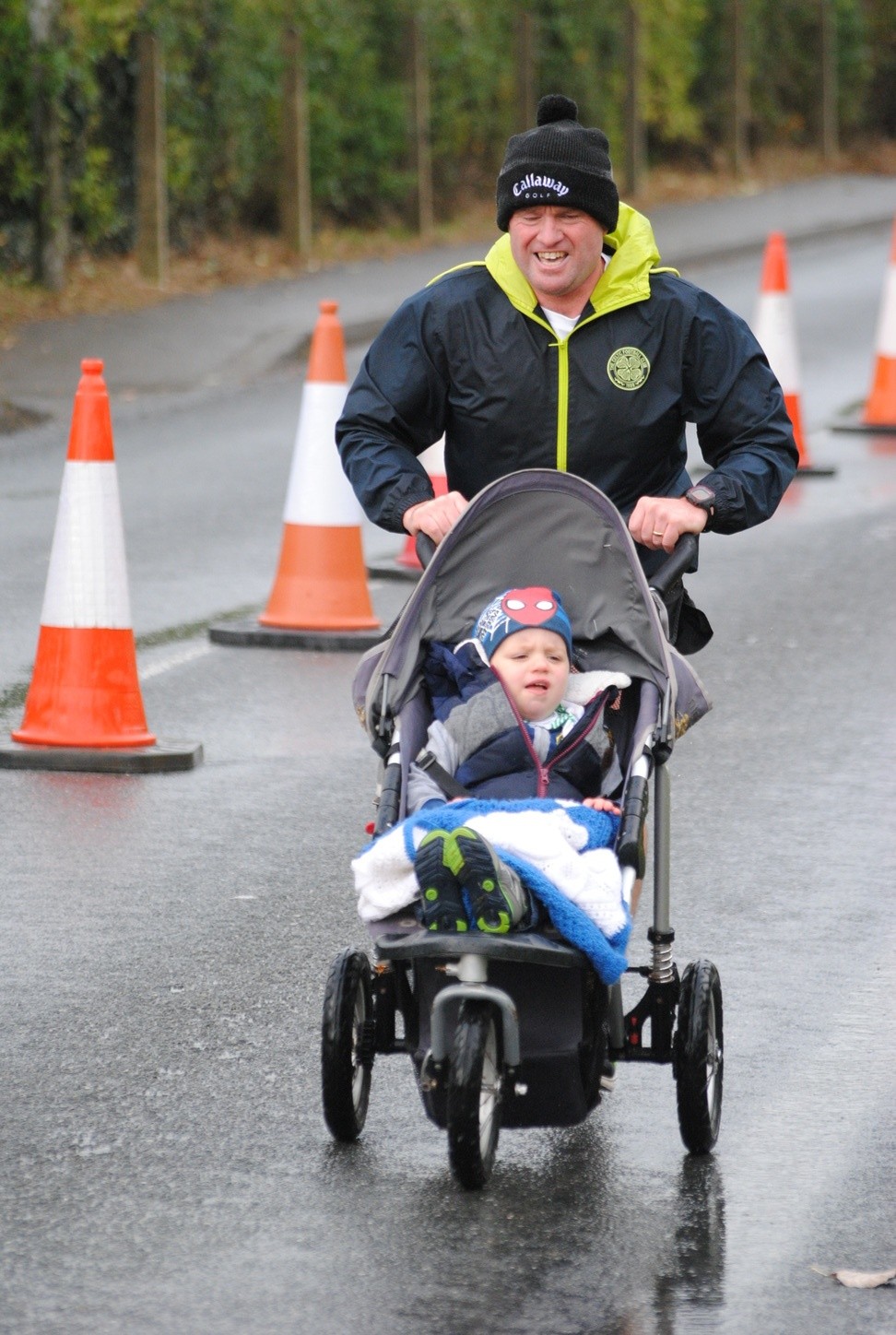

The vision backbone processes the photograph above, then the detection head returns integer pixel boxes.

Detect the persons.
[413,585,627,935]
[336,127,801,650]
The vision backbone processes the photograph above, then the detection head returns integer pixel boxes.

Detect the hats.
[496,94,619,235]
[472,589,574,668]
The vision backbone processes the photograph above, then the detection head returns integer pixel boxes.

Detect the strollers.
[320,464,729,1189]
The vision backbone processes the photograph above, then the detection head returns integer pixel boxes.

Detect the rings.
[653,532,664,536]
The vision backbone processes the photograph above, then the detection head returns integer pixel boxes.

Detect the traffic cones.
[364,432,449,585]
[688,228,838,478]
[823,204,896,435]
[206,298,391,655]
[0,354,205,776]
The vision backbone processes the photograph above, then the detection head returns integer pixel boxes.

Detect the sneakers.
[414,827,468,932]
[446,827,529,933]
